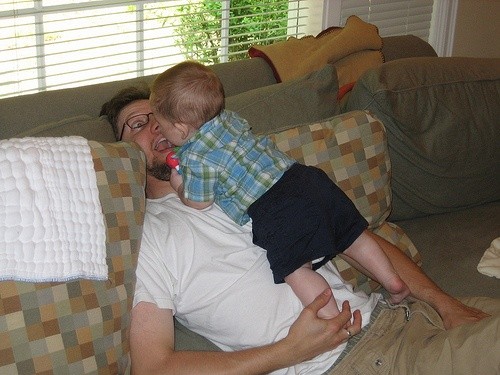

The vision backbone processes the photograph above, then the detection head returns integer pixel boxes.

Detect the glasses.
[120,113,152,140]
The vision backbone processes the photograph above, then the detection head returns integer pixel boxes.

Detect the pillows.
[0,56,500,375]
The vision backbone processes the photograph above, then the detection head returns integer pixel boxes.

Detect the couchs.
[0,34,500,375]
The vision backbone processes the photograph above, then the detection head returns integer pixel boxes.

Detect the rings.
[345,328,353,339]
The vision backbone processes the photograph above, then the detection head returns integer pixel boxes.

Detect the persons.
[100,86,500,375]
[148,61,411,329]
